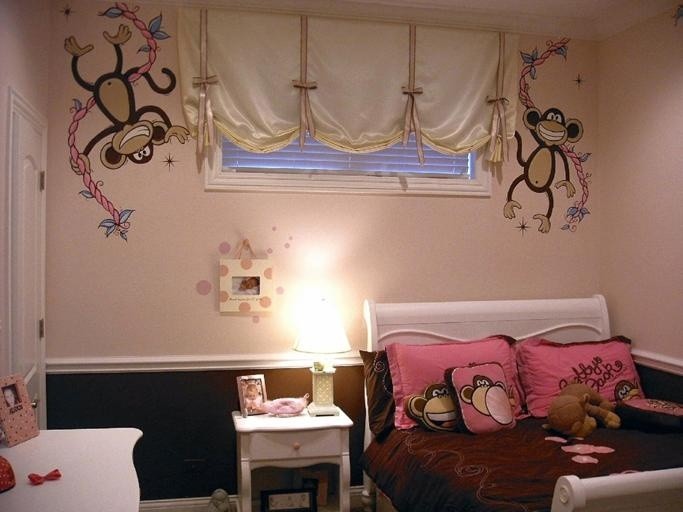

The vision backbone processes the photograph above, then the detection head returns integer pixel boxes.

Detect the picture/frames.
[218,257,275,313]
[235,373,267,416]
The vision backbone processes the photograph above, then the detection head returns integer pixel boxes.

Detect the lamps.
[293,300,350,416]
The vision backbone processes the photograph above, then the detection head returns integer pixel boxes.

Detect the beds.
[361,294,683,512]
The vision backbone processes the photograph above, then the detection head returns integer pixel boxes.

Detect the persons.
[230,276,259,295]
[243,382,263,412]
[4,387,19,406]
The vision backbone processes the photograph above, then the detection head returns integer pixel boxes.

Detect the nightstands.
[230,405,353,511]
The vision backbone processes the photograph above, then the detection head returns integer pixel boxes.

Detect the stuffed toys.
[540,382,621,440]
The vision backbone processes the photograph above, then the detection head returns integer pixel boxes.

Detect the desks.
[0,427,144,511]
